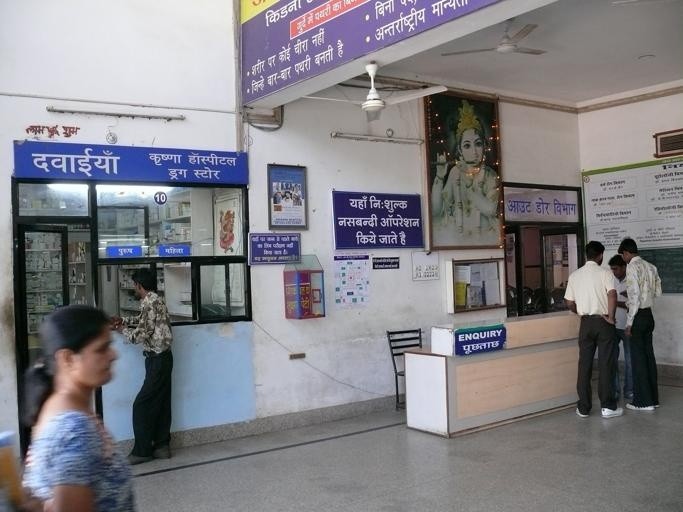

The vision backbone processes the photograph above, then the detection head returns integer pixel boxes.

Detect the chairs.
[385,327,422,410]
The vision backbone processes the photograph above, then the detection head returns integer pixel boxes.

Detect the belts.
[143,349,170,358]
[581,314,605,319]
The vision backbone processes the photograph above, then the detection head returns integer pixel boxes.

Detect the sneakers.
[614,391,621,401]
[624,403,661,412]
[152,443,172,460]
[601,407,624,419]
[576,407,589,418]
[121,453,154,466]
[624,390,634,400]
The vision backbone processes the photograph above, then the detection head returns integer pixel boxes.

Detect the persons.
[605,254,636,401]
[14,302,139,512]
[108,266,175,467]
[615,236,663,413]
[429,99,500,247]
[272,182,302,212]
[561,240,625,418]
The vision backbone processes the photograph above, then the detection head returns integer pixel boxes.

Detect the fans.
[300,61,450,122]
[439,17,546,56]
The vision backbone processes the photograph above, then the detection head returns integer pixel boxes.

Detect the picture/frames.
[417,85,506,252]
[266,163,308,231]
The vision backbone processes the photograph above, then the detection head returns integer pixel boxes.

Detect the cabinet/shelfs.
[115,186,163,333]
[18,204,115,335]
[164,188,213,319]
